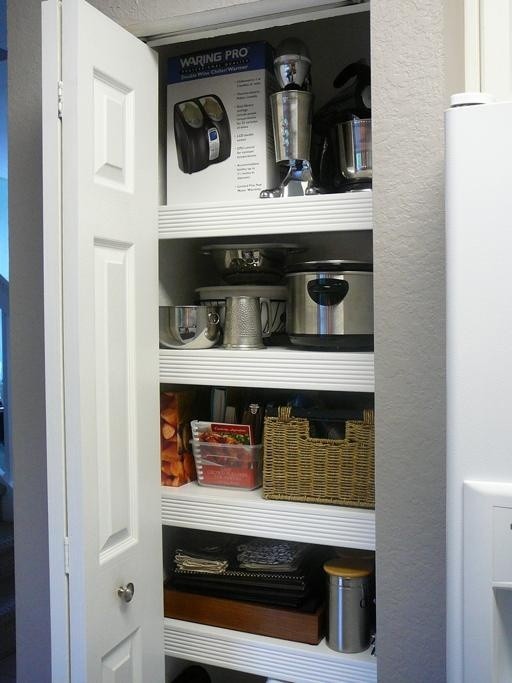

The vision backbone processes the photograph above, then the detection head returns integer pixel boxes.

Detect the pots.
[198,242,309,284]
[337,115,372,192]
[159,305,224,349]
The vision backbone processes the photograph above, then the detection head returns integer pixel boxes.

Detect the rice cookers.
[282,259,374,352]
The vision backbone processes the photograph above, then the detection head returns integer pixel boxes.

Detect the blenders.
[258,35,333,198]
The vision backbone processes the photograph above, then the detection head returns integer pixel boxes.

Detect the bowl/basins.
[193,285,291,344]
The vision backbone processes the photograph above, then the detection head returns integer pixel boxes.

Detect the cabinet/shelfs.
[41,0,377,683]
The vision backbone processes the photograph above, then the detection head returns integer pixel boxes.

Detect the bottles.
[323,558,372,653]
[335,548,375,581]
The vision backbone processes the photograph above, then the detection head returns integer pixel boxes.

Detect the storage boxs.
[167,41,289,205]
[189,439,263,491]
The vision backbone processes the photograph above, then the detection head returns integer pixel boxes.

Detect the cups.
[211,387,240,424]
[226,295,274,350]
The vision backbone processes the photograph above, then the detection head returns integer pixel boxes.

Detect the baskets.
[262,404,374,507]
